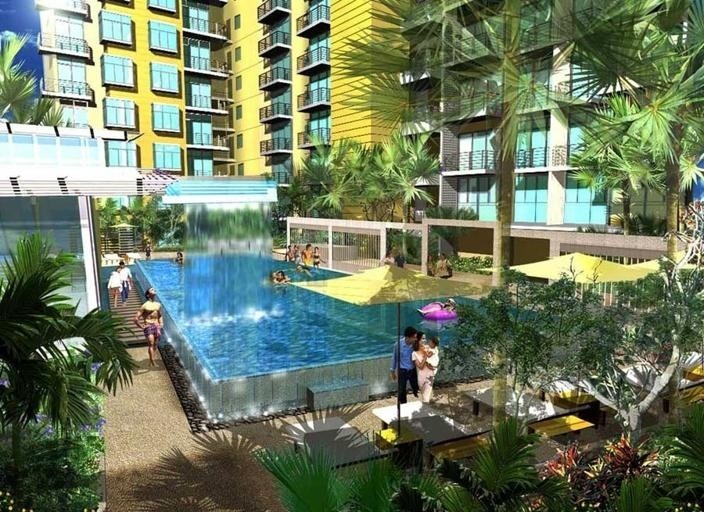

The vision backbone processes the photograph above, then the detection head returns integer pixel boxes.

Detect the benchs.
[526,414,594,439]
[426,435,492,470]
[374,427,422,463]
[554,389,600,434]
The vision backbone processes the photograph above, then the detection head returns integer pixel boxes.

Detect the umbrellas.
[475,250,704,387]
[286,265,496,438]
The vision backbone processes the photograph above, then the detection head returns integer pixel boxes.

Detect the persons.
[382,250,406,267]
[269,244,326,287]
[134,287,163,368]
[435,253,452,280]
[443,298,457,312]
[144,244,152,261]
[175,252,183,264]
[107,262,132,308]
[390,326,441,404]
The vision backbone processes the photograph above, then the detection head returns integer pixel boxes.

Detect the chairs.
[612,344,703,420]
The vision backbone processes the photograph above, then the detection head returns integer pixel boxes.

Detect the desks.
[288,416,392,471]
[372,401,492,447]
[540,376,600,401]
[464,384,567,425]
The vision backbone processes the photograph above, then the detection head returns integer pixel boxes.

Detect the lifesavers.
[421,301,457,319]
